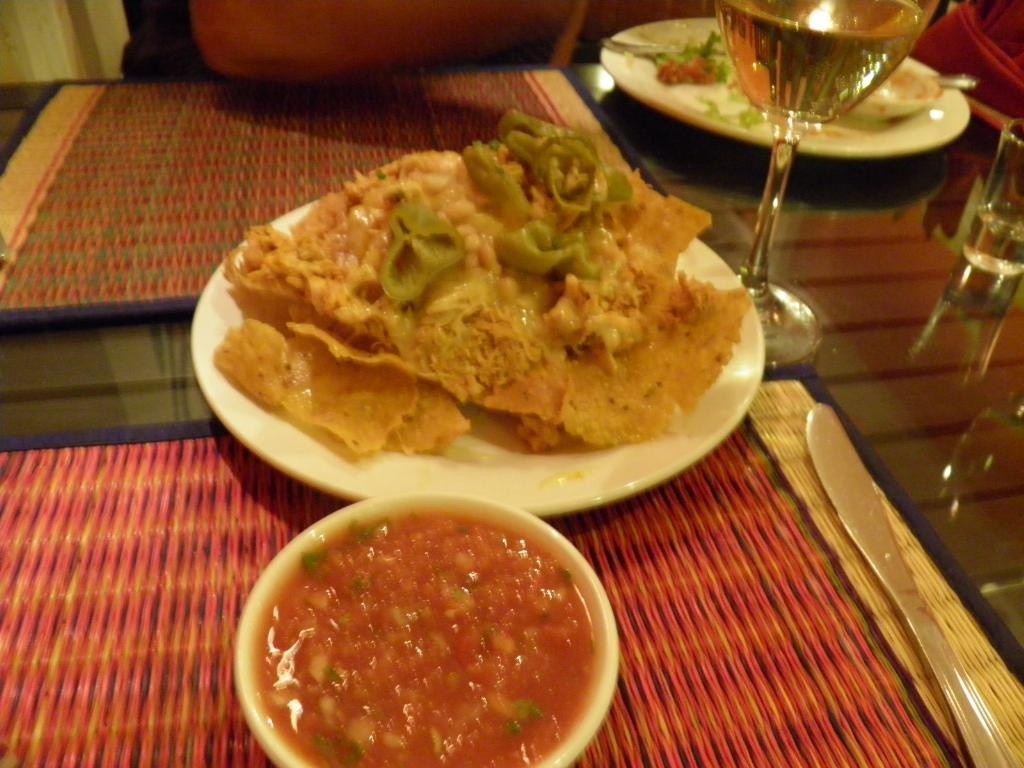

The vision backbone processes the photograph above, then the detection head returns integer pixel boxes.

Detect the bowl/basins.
[232,488,621,768]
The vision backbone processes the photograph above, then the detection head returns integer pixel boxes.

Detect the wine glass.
[713,0,944,372]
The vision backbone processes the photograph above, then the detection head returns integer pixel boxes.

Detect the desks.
[1,67,1024,768]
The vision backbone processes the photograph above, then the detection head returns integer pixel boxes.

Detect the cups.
[958,117,1024,277]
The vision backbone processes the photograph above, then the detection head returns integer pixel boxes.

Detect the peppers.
[377,107,633,303]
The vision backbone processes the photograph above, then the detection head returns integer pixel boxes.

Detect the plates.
[189,200,768,517]
[598,16,971,159]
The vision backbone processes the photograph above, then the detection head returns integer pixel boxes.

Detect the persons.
[189,0,676,83]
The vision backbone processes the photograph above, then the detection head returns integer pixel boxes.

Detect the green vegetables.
[654,30,765,131]
[301,525,571,764]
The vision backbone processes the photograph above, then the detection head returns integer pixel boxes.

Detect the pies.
[214,144,752,455]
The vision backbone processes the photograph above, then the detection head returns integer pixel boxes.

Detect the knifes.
[806,402,1016,767]
